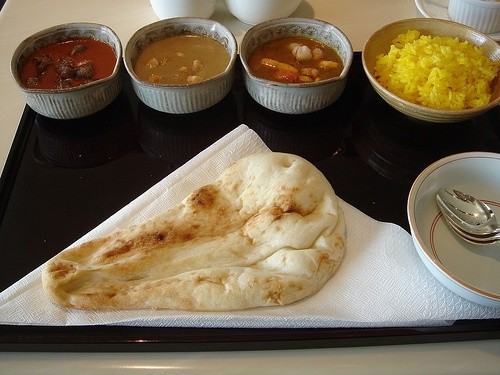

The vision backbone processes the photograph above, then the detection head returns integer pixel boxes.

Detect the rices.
[374,29,499,111]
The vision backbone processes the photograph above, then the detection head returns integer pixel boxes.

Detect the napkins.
[0,124,500,327]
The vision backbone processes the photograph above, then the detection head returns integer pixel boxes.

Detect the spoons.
[436,188,500,247]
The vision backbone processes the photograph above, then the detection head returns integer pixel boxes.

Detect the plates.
[414,0,500,41]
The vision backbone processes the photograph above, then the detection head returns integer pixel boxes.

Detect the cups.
[448,0,500,34]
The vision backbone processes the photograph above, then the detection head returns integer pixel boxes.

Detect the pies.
[42,152,346,313]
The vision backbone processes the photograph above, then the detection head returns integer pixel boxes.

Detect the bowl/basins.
[149,0,215,21]
[239,18,353,114]
[362,18,500,123]
[10,22,123,120]
[123,16,238,113]
[224,0,303,26]
[407,152,500,309]
